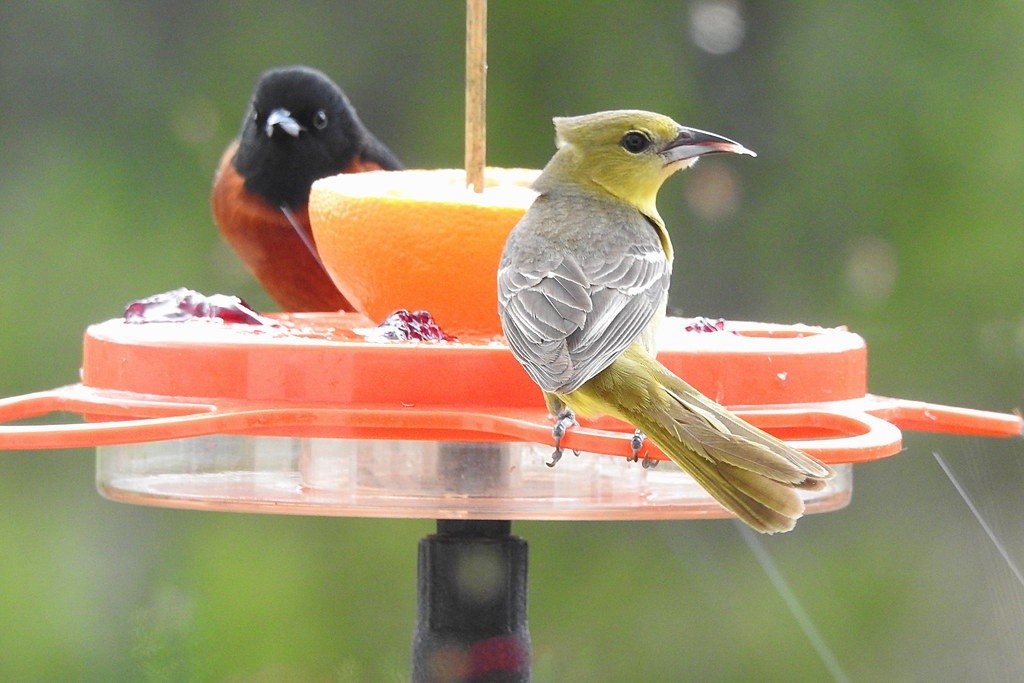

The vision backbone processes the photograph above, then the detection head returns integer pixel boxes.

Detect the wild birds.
[496,109,837,538]
[211,64,407,315]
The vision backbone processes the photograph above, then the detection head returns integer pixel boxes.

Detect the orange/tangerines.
[307,168,540,340]
